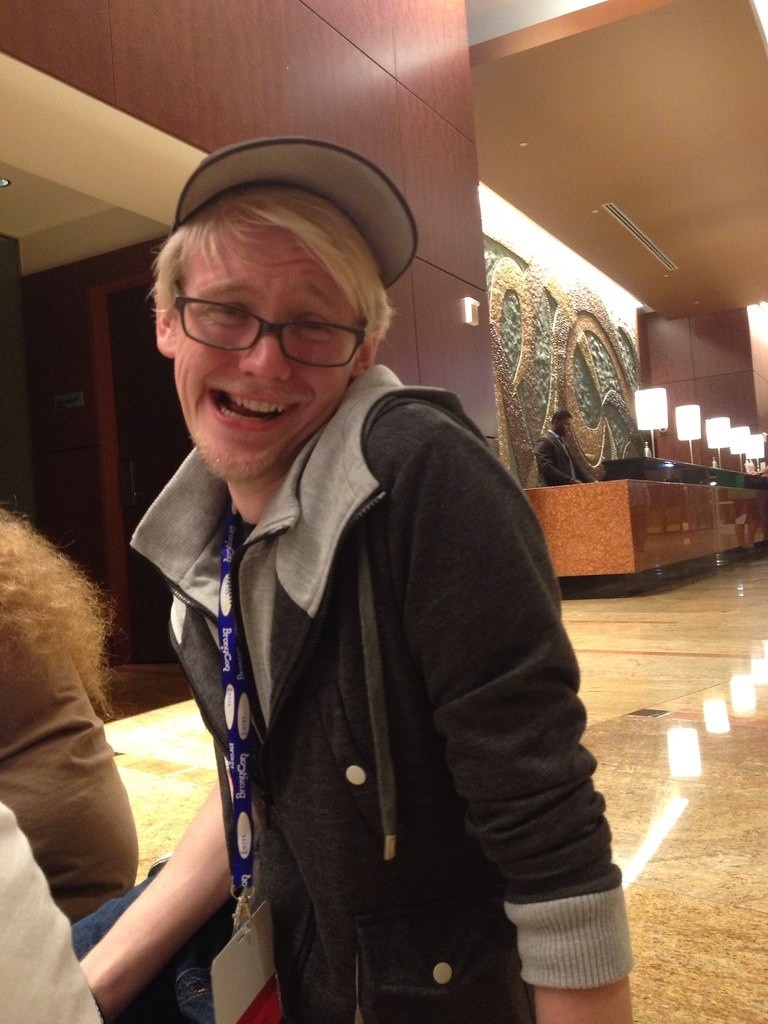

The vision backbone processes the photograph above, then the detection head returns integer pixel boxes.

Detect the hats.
[171,136,420,291]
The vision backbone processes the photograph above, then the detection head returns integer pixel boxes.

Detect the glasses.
[171,279,368,368]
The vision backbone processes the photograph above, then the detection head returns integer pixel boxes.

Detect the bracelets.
[759,471,762,476]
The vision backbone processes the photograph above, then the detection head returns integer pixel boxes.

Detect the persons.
[748,464,768,477]
[728,488,760,551]
[67,135,633,1024]
[1,507,139,1024]
[534,408,598,488]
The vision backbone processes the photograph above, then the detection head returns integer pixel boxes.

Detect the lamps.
[746,434,765,472]
[705,416,732,469]
[730,426,751,474]
[634,387,669,457]
[675,404,701,463]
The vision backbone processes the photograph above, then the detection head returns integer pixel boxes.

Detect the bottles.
[642,442,652,457]
[711,457,717,467]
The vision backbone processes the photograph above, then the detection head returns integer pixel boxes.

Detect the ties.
[558,437,563,443]
